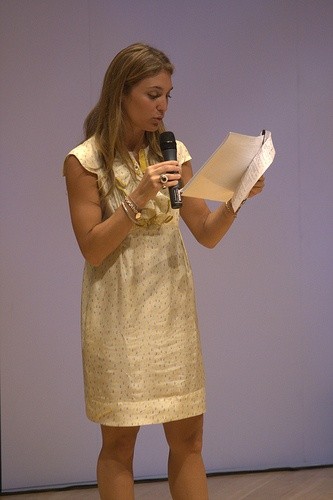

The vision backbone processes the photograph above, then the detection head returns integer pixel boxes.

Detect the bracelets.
[119,194,146,226]
[226,201,238,219]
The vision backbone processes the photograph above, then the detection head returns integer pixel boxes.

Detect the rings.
[162,183,166,189]
[160,173,169,185]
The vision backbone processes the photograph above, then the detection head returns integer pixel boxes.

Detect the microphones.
[159,131,183,209]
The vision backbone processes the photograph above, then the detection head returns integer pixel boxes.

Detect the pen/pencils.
[261,129,267,147]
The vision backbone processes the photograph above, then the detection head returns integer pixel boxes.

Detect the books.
[178,129,277,212]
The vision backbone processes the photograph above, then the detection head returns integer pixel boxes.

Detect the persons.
[61,42,265,500]
